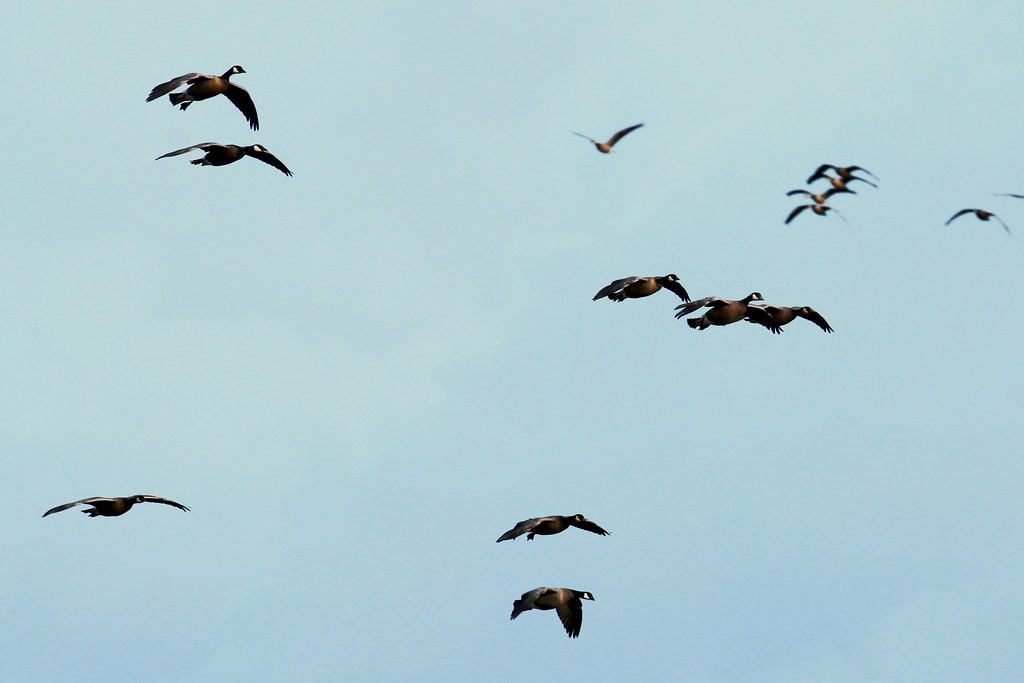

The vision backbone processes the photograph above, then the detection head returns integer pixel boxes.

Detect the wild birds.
[945,209,1011,235]
[785,163,880,227]
[42,495,191,522]
[145,65,260,131]
[744,303,834,335]
[511,587,595,639]
[674,292,765,331]
[496,513,611,543]
[155,142,295,177]
[574,124,645,153]
[591,274,692,304]
